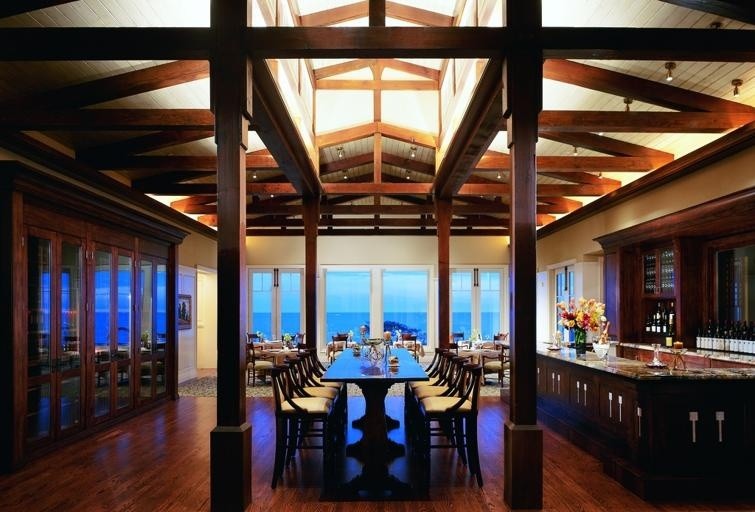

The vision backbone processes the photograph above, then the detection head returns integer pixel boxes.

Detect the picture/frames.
[178,294,192,331]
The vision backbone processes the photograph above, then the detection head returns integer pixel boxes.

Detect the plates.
[646,363,667,367]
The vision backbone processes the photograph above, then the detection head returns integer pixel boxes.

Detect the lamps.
[473,268,479,287]
[731,79,744,97]
[248,142,604,199]
[273,268,279,288]
[623,97,634,112]
[664,61,676,82]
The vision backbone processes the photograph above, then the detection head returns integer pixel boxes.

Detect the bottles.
[646,302,675,335]
[665,326,673,349]
[695,320,754,356]
[644,249,673,297]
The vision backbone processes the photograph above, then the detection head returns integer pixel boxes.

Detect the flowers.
[555,296,608,344]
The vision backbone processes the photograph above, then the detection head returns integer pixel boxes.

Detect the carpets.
[176,361,512,397]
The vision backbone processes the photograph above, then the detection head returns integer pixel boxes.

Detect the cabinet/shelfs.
[639,242,701,299]
[622,346,755,368]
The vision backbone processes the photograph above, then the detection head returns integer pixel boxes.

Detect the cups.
[652,343,661,366]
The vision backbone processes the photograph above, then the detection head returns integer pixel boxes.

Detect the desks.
[319,346,429,504]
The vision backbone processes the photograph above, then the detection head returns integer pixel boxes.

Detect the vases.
[574,327,586,355]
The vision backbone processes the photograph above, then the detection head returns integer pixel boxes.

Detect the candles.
[674,341,682,350]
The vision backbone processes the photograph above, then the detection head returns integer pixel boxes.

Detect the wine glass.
[593,336,611,368]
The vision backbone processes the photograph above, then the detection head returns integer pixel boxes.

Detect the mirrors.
[712,244,754,328]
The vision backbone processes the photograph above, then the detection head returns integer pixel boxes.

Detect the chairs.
[246,332,510,387]
[268,343,350,489]
[404,347,484,490]
